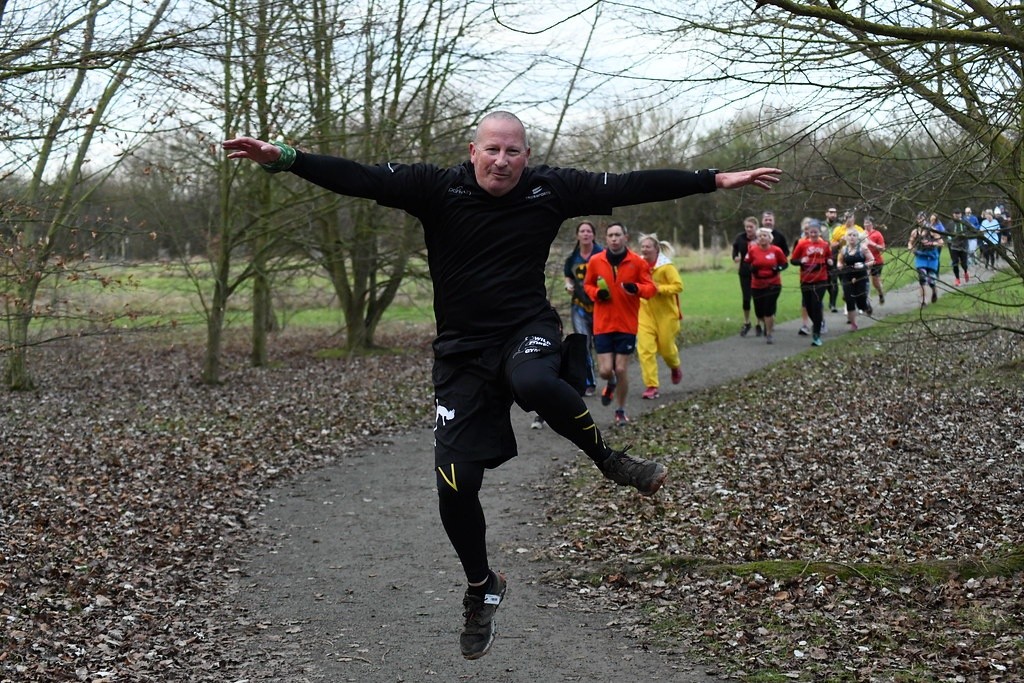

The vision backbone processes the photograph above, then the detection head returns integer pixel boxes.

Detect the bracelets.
[258,141,296,174]
[565,283,574,291]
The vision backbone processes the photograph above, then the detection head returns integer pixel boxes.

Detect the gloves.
[623,282,638,294]
[596,289,609,301]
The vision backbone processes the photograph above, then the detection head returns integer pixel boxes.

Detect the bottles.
[597,276,611,298]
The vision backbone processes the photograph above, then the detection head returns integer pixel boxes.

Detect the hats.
[994,207,1001,215]
[965,207,972,213]
[953,207,961,213]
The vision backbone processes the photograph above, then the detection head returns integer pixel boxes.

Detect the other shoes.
[585,386,595,397]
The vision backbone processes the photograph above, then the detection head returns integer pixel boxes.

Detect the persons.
[759,204,1013,337]
[637,233,684,400]
[563,222,604,396]
[732,217,763,337]
[220,110,783,660]
[744,227,789,344]
[583,222,658,427]
[789,219,835,346]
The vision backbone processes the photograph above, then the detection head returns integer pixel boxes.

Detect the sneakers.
[458,573,506,658]
[530,413,542,430]
[641,386,659,400]
[593,446,668,497]
[670,367,681,384]
[740,270,971,346]
[614,411,628,425]
[601,383,617,406]
